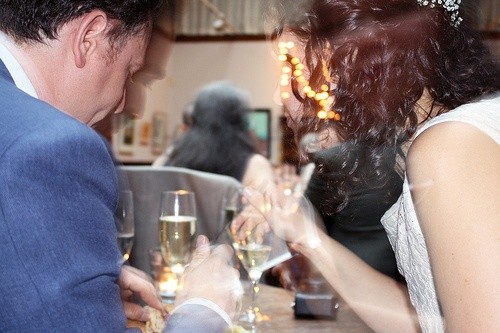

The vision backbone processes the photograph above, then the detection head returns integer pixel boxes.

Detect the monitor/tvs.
[245,108,272,159]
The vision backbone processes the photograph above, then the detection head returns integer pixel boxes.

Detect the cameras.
[291,279,340,318]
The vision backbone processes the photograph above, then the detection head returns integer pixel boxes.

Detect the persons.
[149,0,499,333]
[0,0,247,332]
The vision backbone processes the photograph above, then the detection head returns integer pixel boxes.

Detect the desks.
[125,278,378,333]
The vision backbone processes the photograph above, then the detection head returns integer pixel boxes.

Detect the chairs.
[116,166,249,281]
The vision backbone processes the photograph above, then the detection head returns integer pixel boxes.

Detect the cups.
[147,250,179,304]
[113,191,135,263]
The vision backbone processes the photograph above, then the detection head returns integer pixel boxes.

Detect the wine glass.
[224,192,273,325]
[160,190,197,293]
